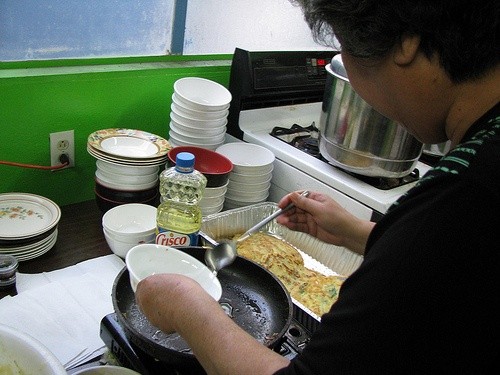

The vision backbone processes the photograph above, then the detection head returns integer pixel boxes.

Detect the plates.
[88,128,171,168]
[0,192,62,262]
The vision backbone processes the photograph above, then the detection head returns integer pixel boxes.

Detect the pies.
[231,232,304,268]
[290,275,349,317]
[266,259,325,293]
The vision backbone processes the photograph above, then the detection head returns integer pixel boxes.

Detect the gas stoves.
[98,299,315,375]
[229,45,441,215]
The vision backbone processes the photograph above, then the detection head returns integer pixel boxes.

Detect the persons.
[136,0,500,375]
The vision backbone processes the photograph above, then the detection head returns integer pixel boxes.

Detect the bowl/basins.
[125,244,223,304]
[169,77,232,152]
[169,140,274,219]
[0,324,68,375]
[95,159,161,215]
[101,203,160,258]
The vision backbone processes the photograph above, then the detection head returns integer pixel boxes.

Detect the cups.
[71,364,143,375]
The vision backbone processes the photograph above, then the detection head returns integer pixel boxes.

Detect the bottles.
[0,254,21,301]
[156,153,208,247]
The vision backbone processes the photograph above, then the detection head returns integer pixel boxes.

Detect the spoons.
[204,191,312,270]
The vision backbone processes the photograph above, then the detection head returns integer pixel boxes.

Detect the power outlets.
[49,130,75,172]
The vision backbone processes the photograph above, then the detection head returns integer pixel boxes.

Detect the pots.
[319,54,425,178]
[112,247,294,362]
